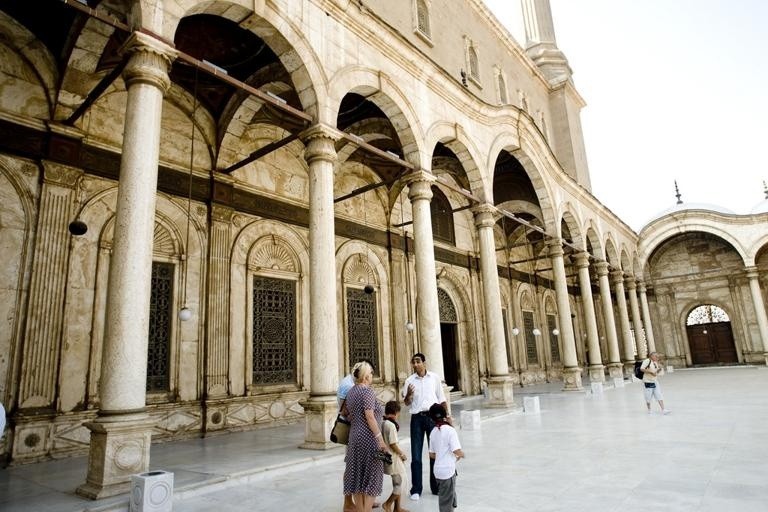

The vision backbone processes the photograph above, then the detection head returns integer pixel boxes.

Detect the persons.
[337,353,465,512]
[640,352,671,415]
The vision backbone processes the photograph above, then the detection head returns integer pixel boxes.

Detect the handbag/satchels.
[330,418,353,445]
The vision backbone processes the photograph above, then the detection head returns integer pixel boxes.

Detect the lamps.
[177,73,200,322]
[399,170,415,332]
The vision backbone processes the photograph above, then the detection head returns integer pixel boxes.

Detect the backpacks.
[634,358,651,380]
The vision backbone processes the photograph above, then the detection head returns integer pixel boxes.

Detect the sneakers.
[663,409,672,415]
[647,408,653,416]
[410,494,421,500]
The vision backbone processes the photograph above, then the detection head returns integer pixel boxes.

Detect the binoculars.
[378,452,392,464]
